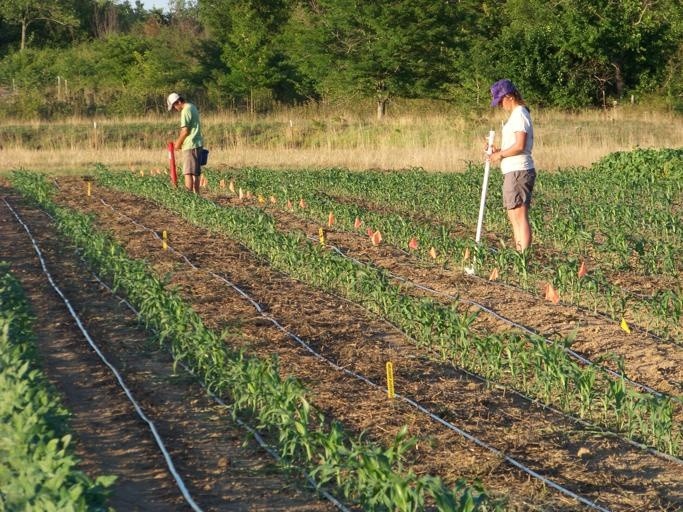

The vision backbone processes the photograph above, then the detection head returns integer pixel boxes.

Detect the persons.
[165,93,209,193]
[485,78,536,253]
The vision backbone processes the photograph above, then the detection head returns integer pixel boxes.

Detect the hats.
[166,92,180,111]
[490,79,514,107]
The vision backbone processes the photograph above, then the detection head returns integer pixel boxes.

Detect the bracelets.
[498,152,503,159]
